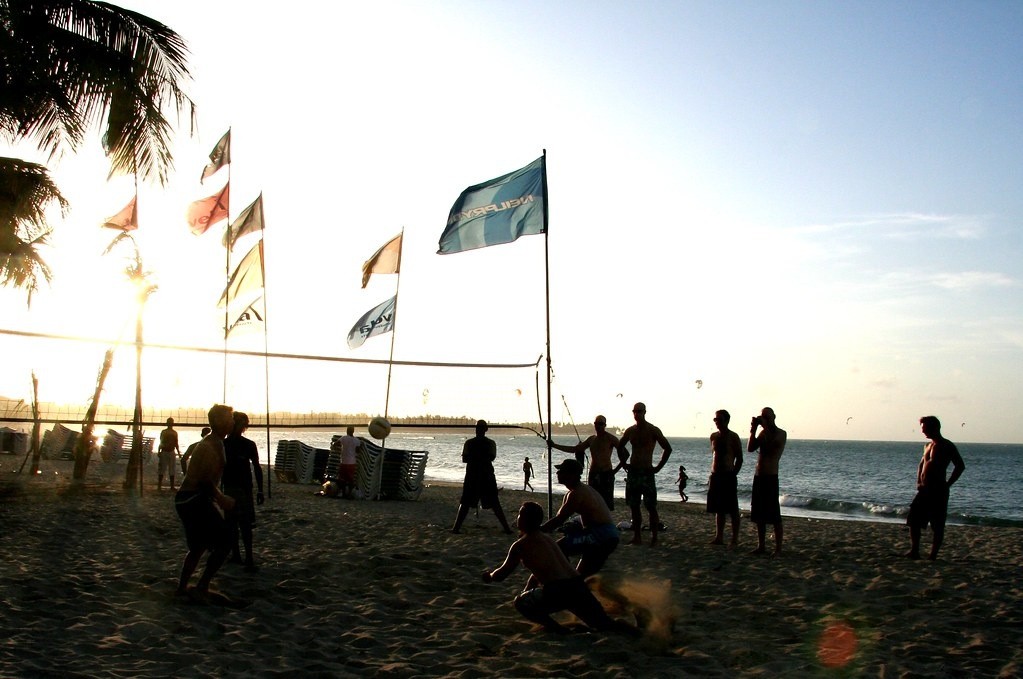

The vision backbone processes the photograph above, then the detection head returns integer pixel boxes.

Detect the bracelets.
[257,491,263,493]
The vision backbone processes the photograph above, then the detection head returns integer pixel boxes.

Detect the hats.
[596,416,606,422]
[633,403,645,412]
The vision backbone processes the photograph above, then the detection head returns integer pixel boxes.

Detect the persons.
[747,408,786,560]
[546,416,630,511]
[618,403,672,545]
[334,427,361,501]
[900,417,966,560]
[481,502,645,641]
[157,405,264,606]
[519,459,652,629]
[523,457,534,492]
[706,410,743,547]
[675,466,689,503]
[447,420,513,535]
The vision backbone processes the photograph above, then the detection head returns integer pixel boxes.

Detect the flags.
[347,295,395,349]
[361,232,403,289]
[436,155,549,255]
[102,193,140,231]
[189,131,267,340]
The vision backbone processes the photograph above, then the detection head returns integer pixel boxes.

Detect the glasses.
[633,410,642,413]
[594,422,605,425]
[714,418,722,422]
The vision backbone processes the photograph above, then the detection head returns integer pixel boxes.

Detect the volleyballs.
[369,418,391,439]
